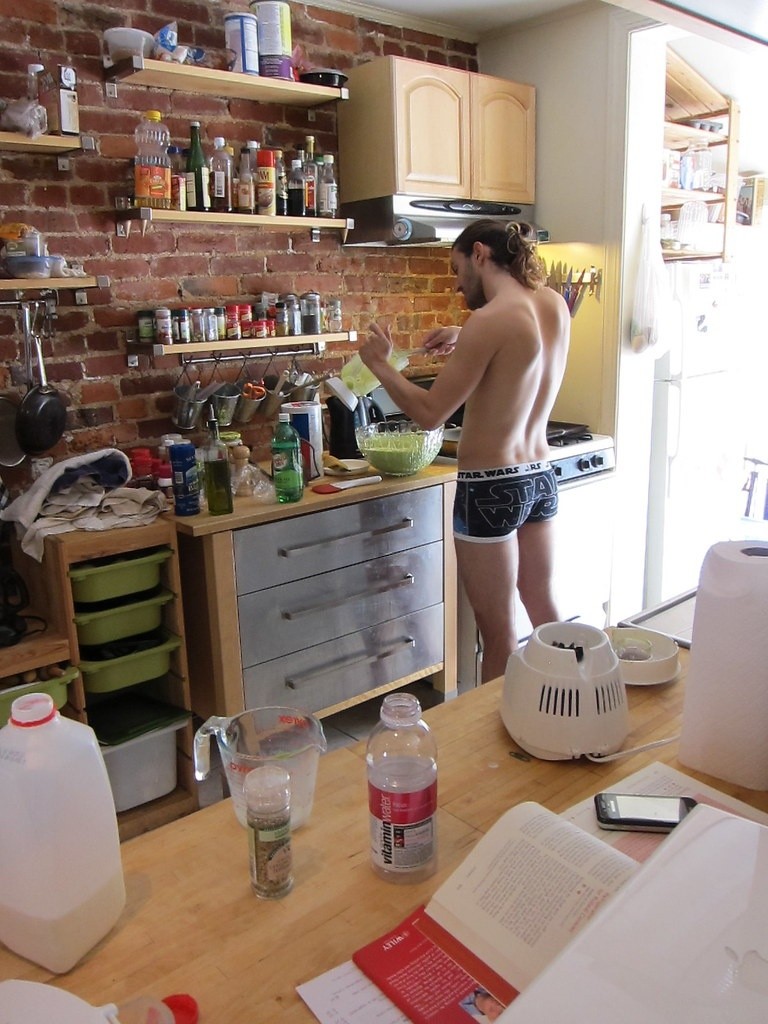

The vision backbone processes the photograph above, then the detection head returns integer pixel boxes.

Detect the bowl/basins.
[355,419,445,478]
[105,26,155,63]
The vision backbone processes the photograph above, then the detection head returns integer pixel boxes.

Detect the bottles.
[203,419,234,514]
[271,413,304,502]
[365,693,437,885]
[115,110,337,220]
[138,292,343,347]
[243,766,294,899]
[131,432,251,514]
[224,0,295,82]
[0,693,127,974]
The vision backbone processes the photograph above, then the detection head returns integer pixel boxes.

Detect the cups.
[172,370,321,430]
[341,342,410,398]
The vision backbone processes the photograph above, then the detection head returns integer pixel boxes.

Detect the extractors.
[341,195,550,248]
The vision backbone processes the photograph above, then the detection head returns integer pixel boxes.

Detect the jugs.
[193,706,329,831]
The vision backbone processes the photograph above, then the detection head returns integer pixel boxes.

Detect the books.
[295,761,768,1024]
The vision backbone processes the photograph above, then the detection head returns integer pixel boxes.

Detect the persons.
[357,219,570,684]
[460,988,506,1024]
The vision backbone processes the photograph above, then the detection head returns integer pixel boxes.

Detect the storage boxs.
[95,721,188,814]
[72,548,173,601]
[0,664,79,731]
[37,64,79,136]
[82,626,181,693]
[77,589,176,646]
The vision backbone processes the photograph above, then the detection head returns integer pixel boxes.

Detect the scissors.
[242,383,265,399]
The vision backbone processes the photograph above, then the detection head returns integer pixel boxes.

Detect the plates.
[299,67,349,87]
[324,459,368,476]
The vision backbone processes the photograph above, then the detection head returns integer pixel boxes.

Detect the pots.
[15,335,66,456]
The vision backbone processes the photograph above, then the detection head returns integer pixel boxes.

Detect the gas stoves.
[367,373,616,483]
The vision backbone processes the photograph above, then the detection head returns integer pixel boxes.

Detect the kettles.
[326,395,388,459]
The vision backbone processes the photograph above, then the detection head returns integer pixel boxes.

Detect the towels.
[0,448,165,565]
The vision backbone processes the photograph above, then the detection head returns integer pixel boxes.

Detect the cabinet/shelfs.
[662,99,744,261]
[95,53,360,358]
[341,53,539,207]
[12,512,207,823]
[0,126,110,304]
[168,461,467,744]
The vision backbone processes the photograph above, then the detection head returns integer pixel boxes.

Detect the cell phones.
[593,794,698,832]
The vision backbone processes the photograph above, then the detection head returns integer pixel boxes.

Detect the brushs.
[311,476,383,494]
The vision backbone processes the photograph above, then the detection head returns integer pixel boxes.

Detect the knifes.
[533,257,586,312]
